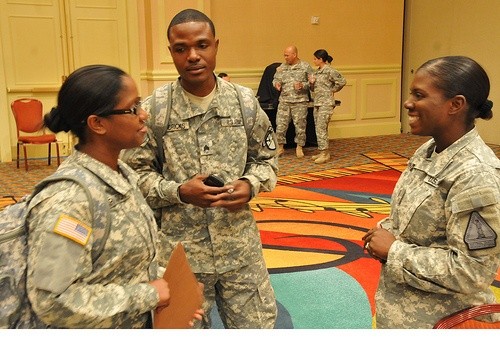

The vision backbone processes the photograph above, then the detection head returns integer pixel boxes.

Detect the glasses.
[81,97,143,125]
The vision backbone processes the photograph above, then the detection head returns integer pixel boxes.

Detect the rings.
[364,242,370,250]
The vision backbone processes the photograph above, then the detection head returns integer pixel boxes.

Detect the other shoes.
[277,144,284,156]
[296,145,304,158]
[314,149,329,164]
[312,151,330,160]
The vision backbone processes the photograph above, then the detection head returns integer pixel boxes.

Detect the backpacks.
[1,168,112,329]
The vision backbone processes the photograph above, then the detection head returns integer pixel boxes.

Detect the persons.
[361,56,500,330]
[19,64,204,330]
[218,73,230,84]
[308,48,348,164]
[116,9,281,329]
[271,47,314,158]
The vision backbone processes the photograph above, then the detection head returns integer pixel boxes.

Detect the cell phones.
[204,174,224,187]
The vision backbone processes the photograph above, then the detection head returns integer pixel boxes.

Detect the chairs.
[11,97,61,172]
